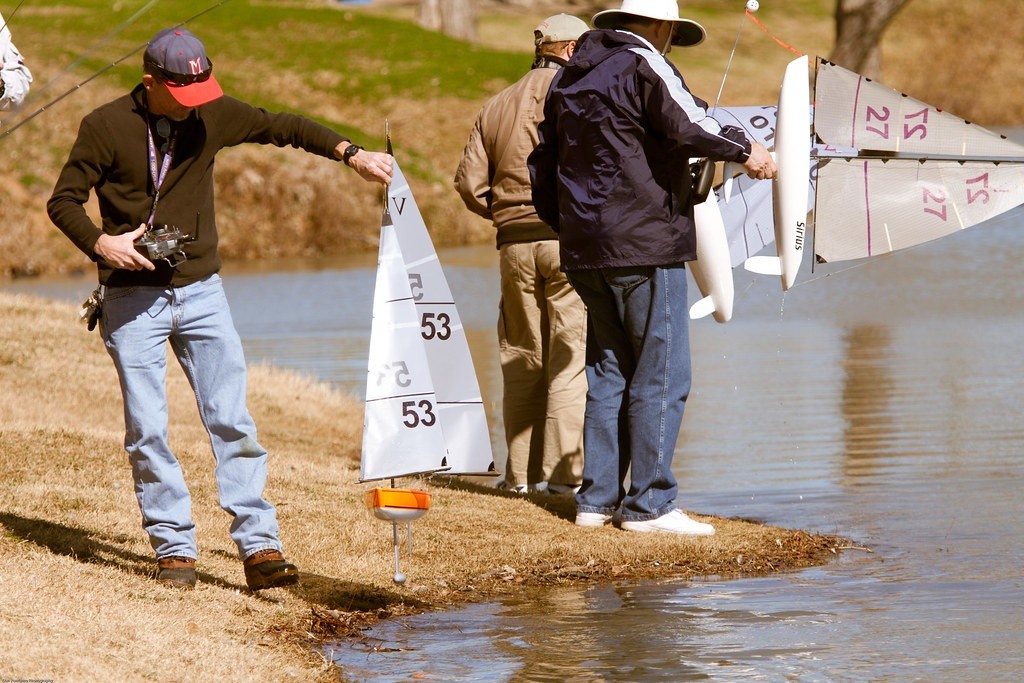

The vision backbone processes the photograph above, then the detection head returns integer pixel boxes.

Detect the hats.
[590,0,706,48]
[534,12,590,47]
[143,27,223,108]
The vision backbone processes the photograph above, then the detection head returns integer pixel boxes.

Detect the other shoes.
[576,504,623,528]
[547,483,581,495]
[153,556,196,591]
[620,508,716,538]
[496,479,526,494]
[245,548,299,591]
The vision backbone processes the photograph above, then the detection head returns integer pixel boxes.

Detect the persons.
[527,0,777,536]
[47,28,395,590]
[451,7,604,502]
[0,5,36,112]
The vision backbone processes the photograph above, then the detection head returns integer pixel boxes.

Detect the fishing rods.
[1,0,228,136]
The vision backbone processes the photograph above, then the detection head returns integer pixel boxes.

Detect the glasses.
[157,58,213,85]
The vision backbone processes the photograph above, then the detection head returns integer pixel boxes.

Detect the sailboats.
[679,105,824,324]
[355,119,501,521]
[767,54,1024,291]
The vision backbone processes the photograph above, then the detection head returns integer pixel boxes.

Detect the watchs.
[342,143,365,167]
